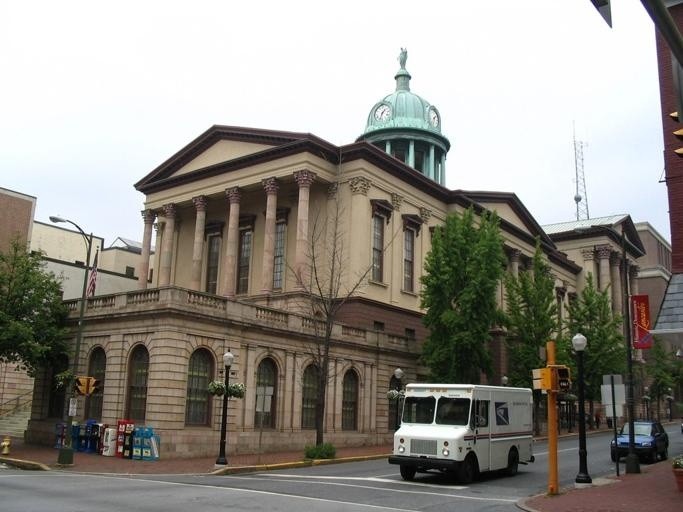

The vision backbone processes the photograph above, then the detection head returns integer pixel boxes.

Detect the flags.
[85,249,97,298]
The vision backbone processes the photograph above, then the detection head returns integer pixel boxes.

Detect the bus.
[388,384,534,483]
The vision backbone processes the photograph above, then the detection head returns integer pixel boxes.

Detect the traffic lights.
[557,368,571,391]
[76,376,100,399]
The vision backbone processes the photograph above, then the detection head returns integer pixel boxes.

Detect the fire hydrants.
[0,436,11,454]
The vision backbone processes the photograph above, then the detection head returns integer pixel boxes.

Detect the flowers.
[54,370,74,392]
[386,390,399,400]
[228,383,246,398]
[207,381,227,397]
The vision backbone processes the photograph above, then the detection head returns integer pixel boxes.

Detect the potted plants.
[672,455,683,495]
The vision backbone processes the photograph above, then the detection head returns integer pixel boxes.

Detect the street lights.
[216,348,234,465]
[572,331,592,483]
[394,367,404,427]
[668,387,672,421]
[644,385,649,420]
[49,215,93,463]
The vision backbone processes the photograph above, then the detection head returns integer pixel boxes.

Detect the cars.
[611,421,669,461]
